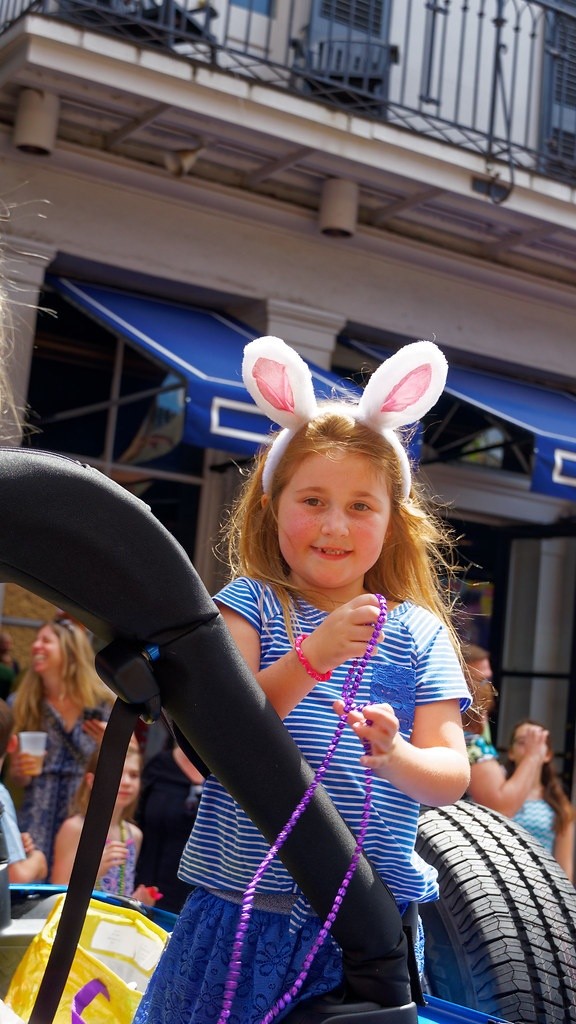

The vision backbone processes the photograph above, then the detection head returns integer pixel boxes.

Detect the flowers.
[131,884,163,906]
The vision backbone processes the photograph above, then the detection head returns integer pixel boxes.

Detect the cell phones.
[83,708,102,733]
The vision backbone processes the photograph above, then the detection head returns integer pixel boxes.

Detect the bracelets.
[295,633,332,681]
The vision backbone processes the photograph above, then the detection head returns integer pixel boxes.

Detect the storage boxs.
[0,919,47,1002]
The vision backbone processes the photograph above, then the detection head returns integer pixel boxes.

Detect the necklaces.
[217,592,387,1024]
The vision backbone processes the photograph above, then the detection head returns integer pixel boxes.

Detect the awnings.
[56,278,422,471]
[342,335,576,501]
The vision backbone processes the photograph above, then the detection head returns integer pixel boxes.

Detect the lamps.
[316,177,359,239]
[162,136,208,177]
[13,89,61,155]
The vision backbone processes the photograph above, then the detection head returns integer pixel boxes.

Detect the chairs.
[287,37,400,117]
[124,0,218,64]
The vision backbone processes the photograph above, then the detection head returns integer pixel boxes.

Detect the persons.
[505,720,574,884]
[0,615,139,915]
[128,745,205,917]
[130,335,474,1024]
[52,742,159,904]
[0,703,48,911]
[460,643,549,818]
